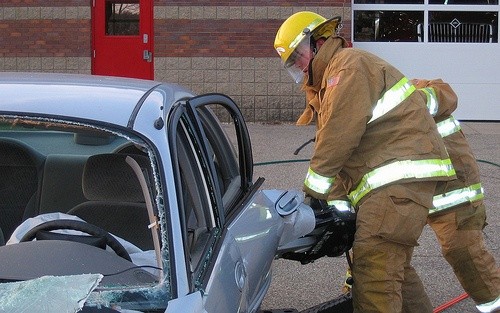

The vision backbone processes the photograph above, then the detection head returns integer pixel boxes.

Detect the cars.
[0,71,285,313]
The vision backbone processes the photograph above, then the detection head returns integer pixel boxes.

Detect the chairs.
[64,153,162,250]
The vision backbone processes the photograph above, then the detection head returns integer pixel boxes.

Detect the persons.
[400,78,500,312]
[273,10,457,313]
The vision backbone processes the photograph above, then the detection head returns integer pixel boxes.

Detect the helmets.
[273,11,342,70]
